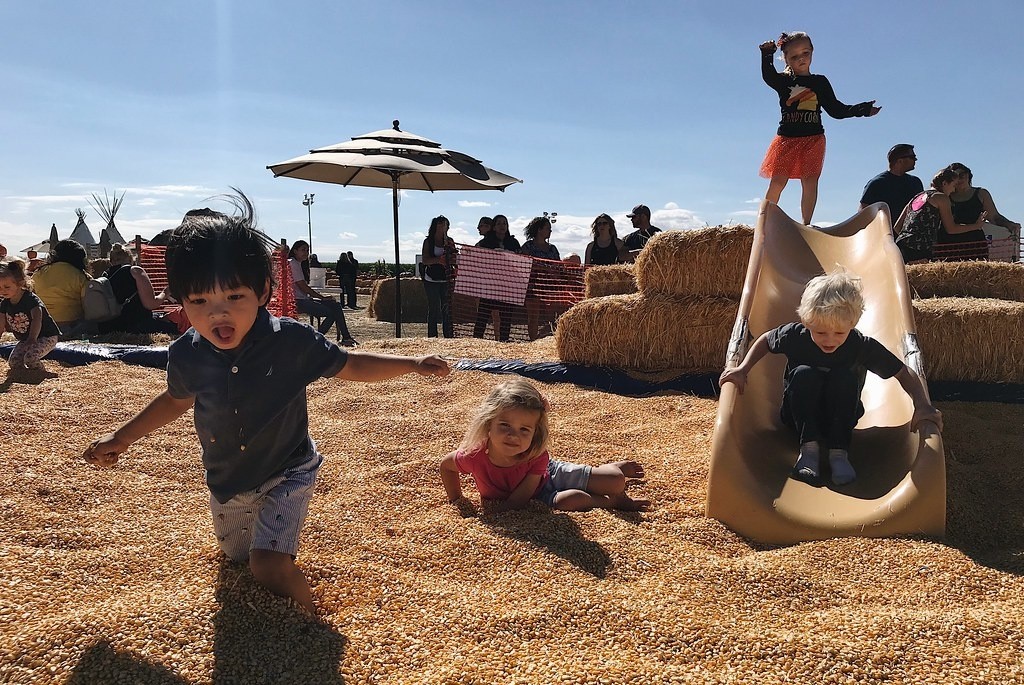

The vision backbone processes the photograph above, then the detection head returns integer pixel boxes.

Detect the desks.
[307,287,342,342]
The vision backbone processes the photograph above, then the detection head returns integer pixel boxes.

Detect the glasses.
[895,152,916,160]
[597,221,608,226]
[478,223,488,227]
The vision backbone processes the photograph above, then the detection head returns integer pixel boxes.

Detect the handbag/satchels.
[430,248,446,256]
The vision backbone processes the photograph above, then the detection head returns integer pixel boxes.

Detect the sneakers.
[342,338,359,347]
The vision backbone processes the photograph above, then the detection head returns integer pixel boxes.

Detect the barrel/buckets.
[309,267,326,290]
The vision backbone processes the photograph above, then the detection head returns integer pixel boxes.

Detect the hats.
[626,205,650,218]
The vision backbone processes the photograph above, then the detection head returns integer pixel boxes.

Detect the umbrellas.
[19,215,127,257]
[265,121,525,339]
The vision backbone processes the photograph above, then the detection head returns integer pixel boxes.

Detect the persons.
[0,260,63,370]
[440,381,645,512]
[859,143,925,261]
[894,170,986,263]
[759,30,881,226]
[0,240,192,335]
[618,204,662,263]
[719,265,943,485]
[939,163,1019,260]
[84,185,450,615]
[272,215,581,346]
[585,213,624,265]
